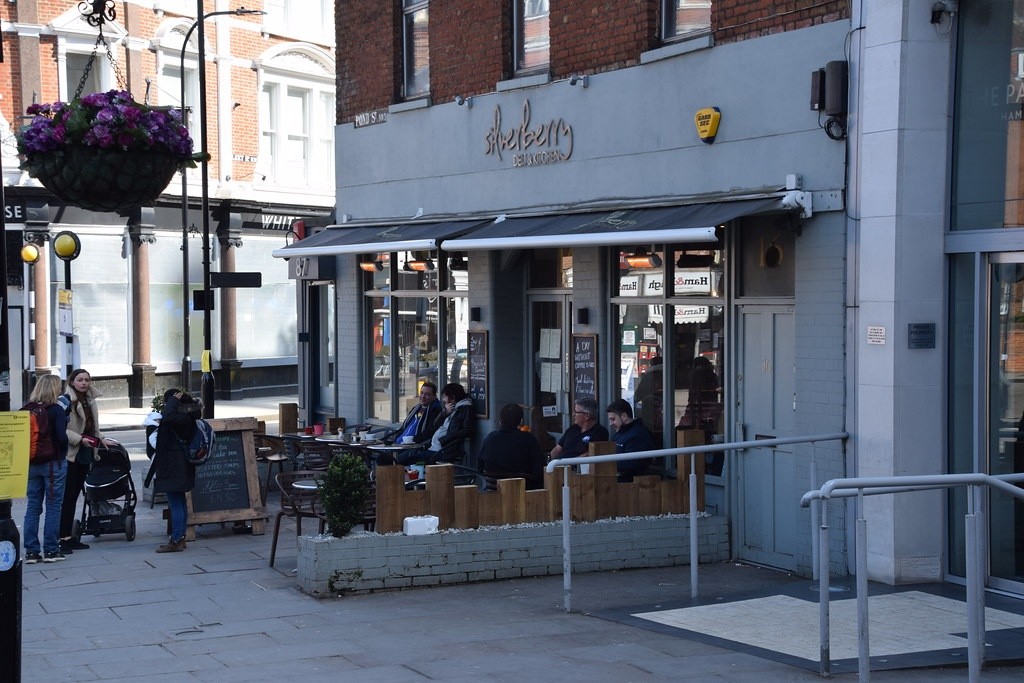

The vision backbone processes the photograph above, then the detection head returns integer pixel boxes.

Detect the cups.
[365,434,376,439]
[306,426,313,435]
[403,436,414,443]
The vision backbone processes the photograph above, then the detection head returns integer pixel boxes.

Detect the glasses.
[574,410,592,416]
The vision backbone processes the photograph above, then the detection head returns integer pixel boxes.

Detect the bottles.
[338,427,344,438]
[352,433,358,444]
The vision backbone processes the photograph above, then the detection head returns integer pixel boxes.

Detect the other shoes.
[71,536,89,549]
[156,534,187,553]
[58,538,72,554]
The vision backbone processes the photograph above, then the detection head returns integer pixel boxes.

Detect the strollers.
[70,438,137,542]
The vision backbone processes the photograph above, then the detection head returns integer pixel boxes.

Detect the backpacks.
[170,413,216,466]
[19,401,61,466]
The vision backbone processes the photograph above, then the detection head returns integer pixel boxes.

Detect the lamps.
[568,74,588,87]
[577,307,589,326]
[359,257,384,271]
[471,306,481,324]
[623,243,663,269]
[402,251,435,271]
[454,94,473,106]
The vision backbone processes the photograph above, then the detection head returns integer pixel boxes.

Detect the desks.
[374,463,453,488]
[335,441,365,458]
[280,429,312,443]
[314,433,351,444]
[366,440,426,464]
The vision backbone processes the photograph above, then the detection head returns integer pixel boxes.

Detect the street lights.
[181,6,267,391]
[20,231,81,379]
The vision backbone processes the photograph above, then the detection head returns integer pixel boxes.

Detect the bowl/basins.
[384,441,395,446]
[408,472,419,479]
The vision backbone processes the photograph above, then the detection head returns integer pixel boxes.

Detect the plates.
[364,439,376,443]
[400,442,415,445]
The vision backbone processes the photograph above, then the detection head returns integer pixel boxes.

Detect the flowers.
[19,89,195,158]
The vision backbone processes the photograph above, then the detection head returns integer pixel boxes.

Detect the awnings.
[440,196,782,251]
[271,216,493,258]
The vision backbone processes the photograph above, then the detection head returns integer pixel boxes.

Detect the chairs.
[252,424,533,568]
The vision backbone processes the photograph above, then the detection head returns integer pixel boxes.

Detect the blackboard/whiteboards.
[184,416,268,524]
[571,334,597,414]
[467,329,488,419]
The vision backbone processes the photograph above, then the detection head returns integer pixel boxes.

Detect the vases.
[35,151,178,212]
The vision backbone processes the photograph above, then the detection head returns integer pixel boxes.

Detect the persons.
[18,368,119,564]
[479,403,548,493]
[606,398,654,482]
[143,389,200,552]
[550,397,608,469]
[688,357,718,403]
[375,381,475,465]
[635,347,664,430]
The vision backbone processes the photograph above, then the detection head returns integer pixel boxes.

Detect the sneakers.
[43,551,66,563]
[25,552,42,564]
